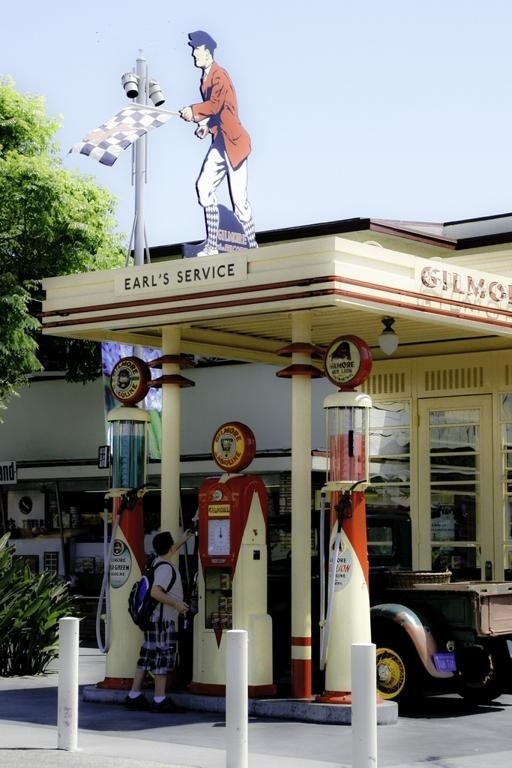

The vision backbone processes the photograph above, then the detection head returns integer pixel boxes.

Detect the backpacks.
[126,560,177,632]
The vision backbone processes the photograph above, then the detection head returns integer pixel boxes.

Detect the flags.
[65,104,176,166]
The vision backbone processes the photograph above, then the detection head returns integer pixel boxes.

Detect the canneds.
[211,572,232,629]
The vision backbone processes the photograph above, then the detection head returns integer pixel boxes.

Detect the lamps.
[120,72,139,99]
[378,317,400,356]
[145,78,166,108]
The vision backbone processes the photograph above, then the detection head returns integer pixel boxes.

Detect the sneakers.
[149,697,186,714]
[122,689,151,712]
[196,245,220,257]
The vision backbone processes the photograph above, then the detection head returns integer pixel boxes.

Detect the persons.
[178,29,261,258]
[124,528,194,714]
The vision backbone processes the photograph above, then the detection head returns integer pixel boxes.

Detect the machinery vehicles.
[266,514,512,702]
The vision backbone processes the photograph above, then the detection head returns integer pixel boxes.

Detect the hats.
[186,28,217,52]
